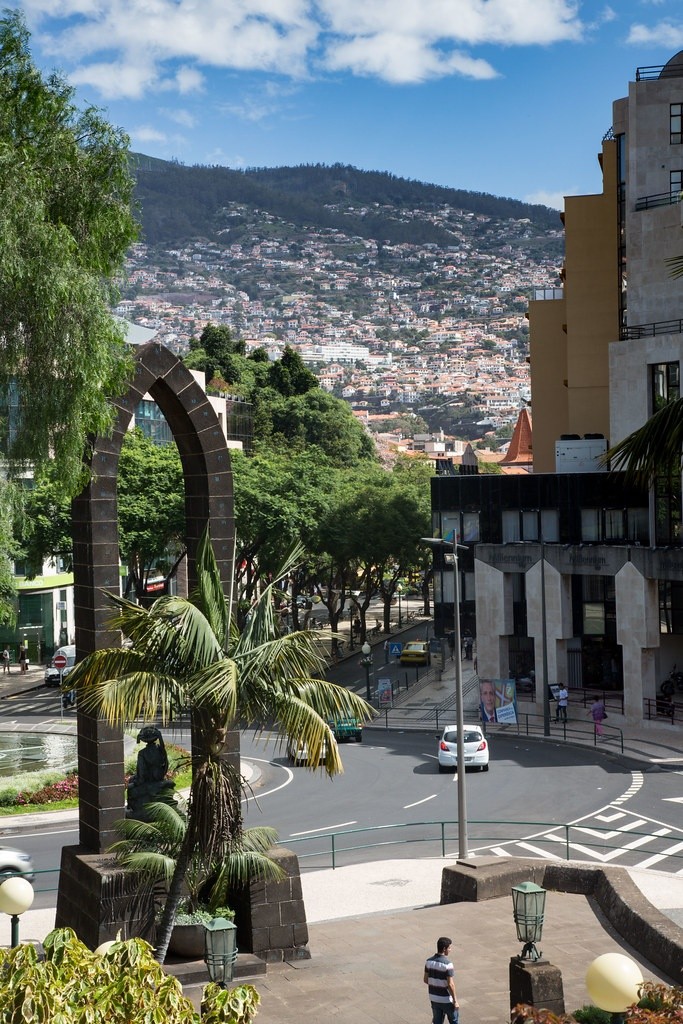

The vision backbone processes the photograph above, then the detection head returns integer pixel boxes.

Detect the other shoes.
[561,720,567,724]
[599,735,602,739]
[553,719,560,722]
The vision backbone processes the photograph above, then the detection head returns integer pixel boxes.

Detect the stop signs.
[54,656,67,669]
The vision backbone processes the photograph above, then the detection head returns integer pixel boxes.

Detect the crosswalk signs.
[389,643,402,656]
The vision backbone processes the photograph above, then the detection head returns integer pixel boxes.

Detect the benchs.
[324,607,423,659]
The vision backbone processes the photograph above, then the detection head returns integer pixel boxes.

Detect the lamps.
[586,952,644,1013]
[203,916,238,991]
[510,881,547,961]
[0,877,34,915]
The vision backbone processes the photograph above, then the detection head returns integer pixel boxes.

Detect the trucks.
[44,645,75,688]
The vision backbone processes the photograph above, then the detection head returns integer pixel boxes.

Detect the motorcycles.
[656,694,675,717]
[660,663,683,695]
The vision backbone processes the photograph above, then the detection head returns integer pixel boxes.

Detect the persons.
[423,937,460,1024]
[481,680,498,722]
[125,727,176,811]
[19,646,26,675]
[3,645,10,675]
[464,637,473,660]
[586,696,606,739]
[556,683,568,723]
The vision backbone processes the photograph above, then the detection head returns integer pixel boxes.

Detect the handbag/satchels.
[603,711,608,719]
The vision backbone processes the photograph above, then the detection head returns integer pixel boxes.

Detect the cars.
[435,723,490,773]
[286,709,365,766]
[400,640,429,666]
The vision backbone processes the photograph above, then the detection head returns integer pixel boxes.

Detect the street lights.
[0,877,34,948]
[420,528,471,866]
[507,532,551,738]
[359,641,375,701]
